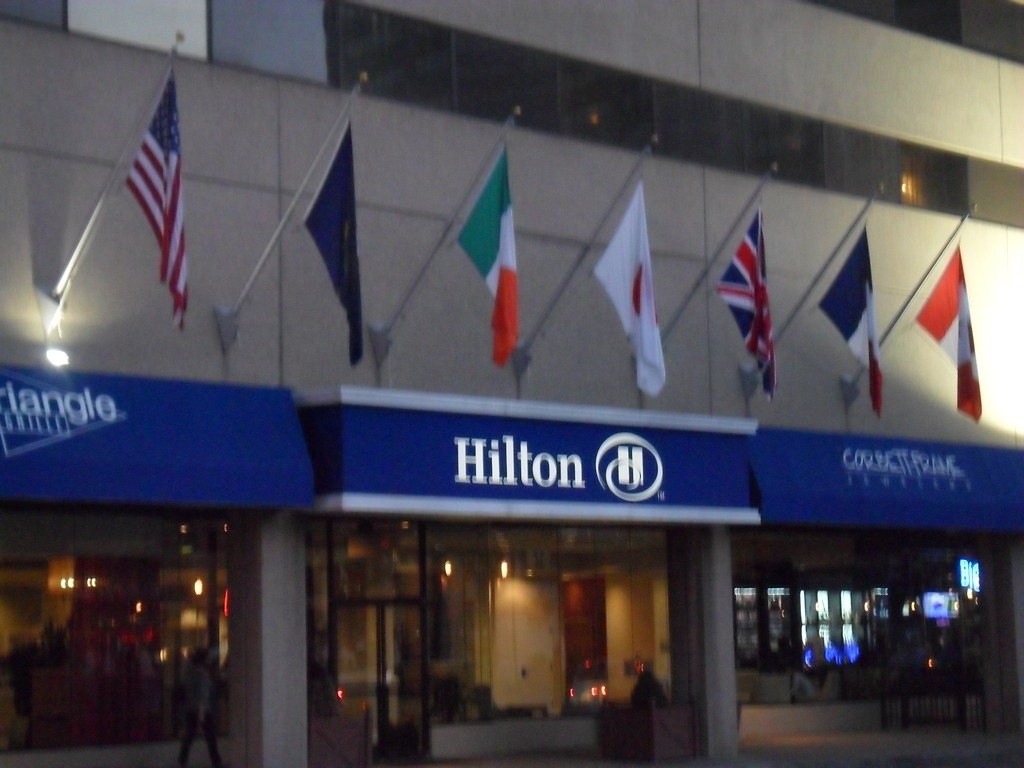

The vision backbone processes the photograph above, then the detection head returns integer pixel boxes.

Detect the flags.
[125,74,191,331]
[715,209,777,402]
[457,147,521,369]
[302,124,363,366]
[917,247,983,424]
[592,178,667,397]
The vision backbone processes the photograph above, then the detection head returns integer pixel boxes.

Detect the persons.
[176,646,227,768]
[820,227,883,421]
